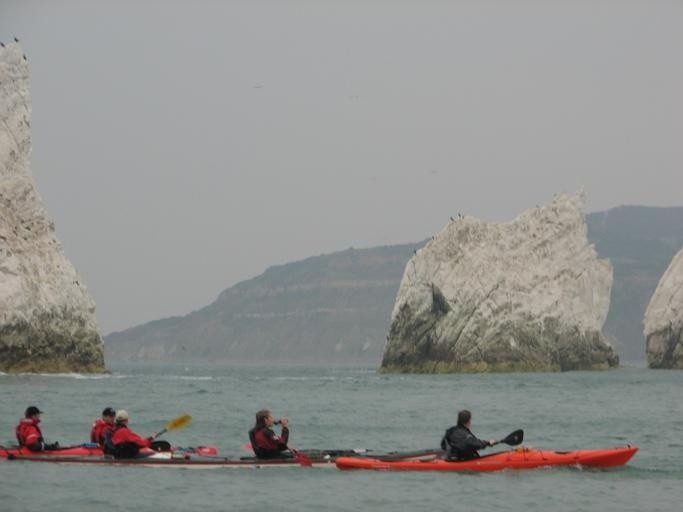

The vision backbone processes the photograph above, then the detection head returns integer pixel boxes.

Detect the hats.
[24,405,45,418]
[114,409,130,421]
[101,406,116,417]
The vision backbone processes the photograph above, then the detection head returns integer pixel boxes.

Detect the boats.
[0,439,223,459]
[332,441,641,476]
[7,447,449,470]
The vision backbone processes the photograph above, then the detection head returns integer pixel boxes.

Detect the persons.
[15,405,101,452]
[91,408,116,446]
[248,409,336,461]
[97,409,171,460]
[442,410,495,462]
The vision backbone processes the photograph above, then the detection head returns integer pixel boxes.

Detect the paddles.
[284,445,313,465]
[491,429,524,446]
[149,414,191,440]
